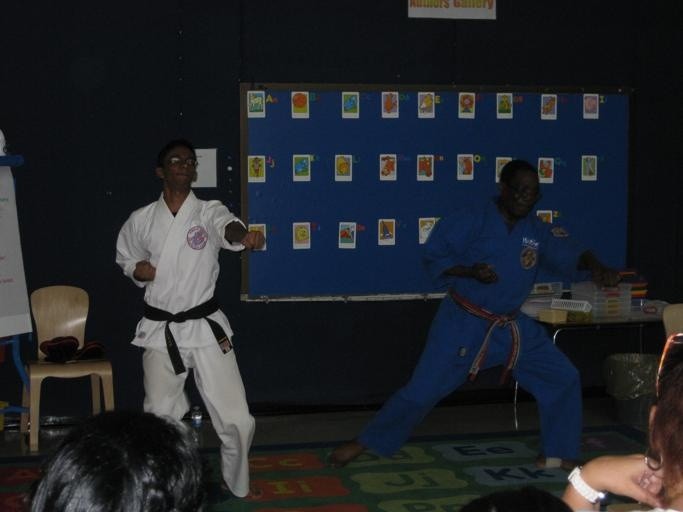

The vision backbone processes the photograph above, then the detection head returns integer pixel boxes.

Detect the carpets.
[0,424,648,512]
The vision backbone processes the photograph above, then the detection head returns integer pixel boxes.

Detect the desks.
[514,298,671,431]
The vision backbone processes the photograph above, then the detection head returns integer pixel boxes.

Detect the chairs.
[657,303,683,344]
[19,284,115,453]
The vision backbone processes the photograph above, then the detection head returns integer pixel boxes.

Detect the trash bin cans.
[607,353,660,434]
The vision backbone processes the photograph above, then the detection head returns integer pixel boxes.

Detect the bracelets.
[567,464,608,505]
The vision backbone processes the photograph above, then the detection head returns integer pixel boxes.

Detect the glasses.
[164,157,197,166]
[656,333,682,397]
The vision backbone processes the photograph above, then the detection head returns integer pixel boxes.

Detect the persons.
[25,410,212,512]
[561,333,683,512]
[457,485,576,512]
[325,157,624,471]
[114,138,268,500]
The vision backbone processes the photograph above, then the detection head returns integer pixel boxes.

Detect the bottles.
[191,406,204,451]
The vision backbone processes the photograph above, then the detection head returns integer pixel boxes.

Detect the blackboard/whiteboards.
[237,83,631,302]
